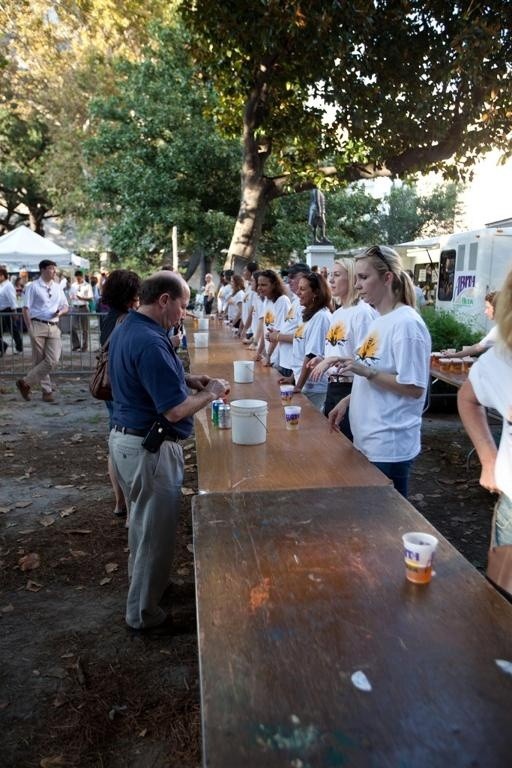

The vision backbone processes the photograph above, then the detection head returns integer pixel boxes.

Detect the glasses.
[309,273,317,280]
[48,288,52,298]
[363,246,391,272]
[288,275,297,279]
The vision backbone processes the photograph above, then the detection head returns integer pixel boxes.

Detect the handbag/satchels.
[89,313,127,401]
[487,543,512,596]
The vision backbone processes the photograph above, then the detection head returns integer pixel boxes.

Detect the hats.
[281,263,311,276]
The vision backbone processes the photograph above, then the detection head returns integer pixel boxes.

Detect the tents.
[0,222,90,282]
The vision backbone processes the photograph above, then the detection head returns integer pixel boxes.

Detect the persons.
[443,270,512,606]
[1,259,107,354]
[93,270,230,637]
[308,189,333,244]
[16,260,69,403]
[205,245,431,498]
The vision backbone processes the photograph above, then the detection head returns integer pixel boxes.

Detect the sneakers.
[42,389,54,401]
[16,379,32,401]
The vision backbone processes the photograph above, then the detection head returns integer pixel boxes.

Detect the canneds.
[210,399,224,424]
[218,404,232,429]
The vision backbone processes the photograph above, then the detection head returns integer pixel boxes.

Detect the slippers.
[112,507,126,517]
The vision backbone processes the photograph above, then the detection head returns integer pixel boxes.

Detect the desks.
[182,314,394,492]
[423,352,504,474]
[192,486,511,768]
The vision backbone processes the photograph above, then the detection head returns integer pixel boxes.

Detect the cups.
[280,385,294,406]
[430,349,474,374]
[232,328,238,336]
[284,405,301,430]
[402,531,437,584]
[246,332,253,339]
[224,320,230,326]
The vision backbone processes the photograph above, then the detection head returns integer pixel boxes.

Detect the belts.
[30,317,57,325]
[329,375,353,383]
[112,424,180,442]
[74,304,86,308]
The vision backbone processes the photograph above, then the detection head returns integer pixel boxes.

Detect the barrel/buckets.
[193,332,209,348]
[198,318,209,330]
[233,361,255,383]
[230,398,268,445]
